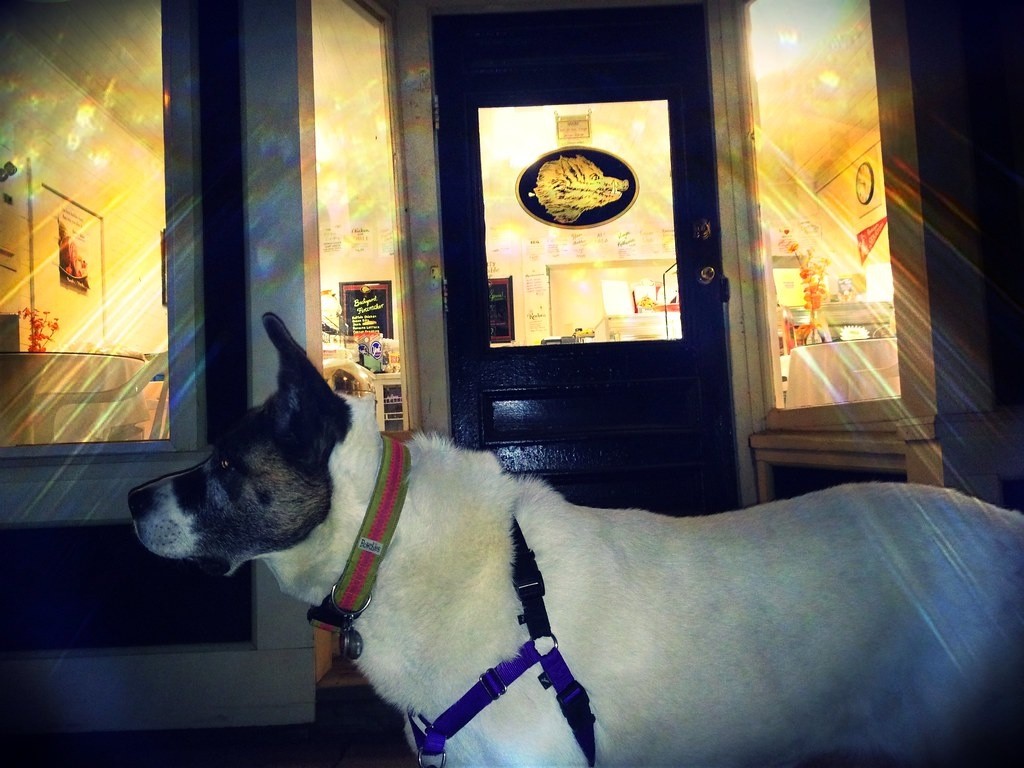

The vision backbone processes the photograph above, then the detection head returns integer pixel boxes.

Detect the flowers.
[15,304,61,353]
[635,295,658,310]
[781,226,831,319]
[839,325,870,341]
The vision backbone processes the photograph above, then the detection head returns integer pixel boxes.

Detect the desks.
[0,349,148,449]
[372,372,402,431]
[785,337,901,408]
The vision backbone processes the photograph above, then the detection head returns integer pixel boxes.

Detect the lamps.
[0,161,18,182]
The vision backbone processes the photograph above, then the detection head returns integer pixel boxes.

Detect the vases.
[806,321,823,345]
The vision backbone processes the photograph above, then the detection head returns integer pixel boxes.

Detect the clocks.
[856,162,875,206]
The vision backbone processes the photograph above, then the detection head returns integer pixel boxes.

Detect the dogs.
[127,310,1024,768]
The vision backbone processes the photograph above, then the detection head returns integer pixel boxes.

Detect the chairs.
[34,350,171,444]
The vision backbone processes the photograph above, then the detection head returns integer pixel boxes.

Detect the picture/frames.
[338,280,393,340]
